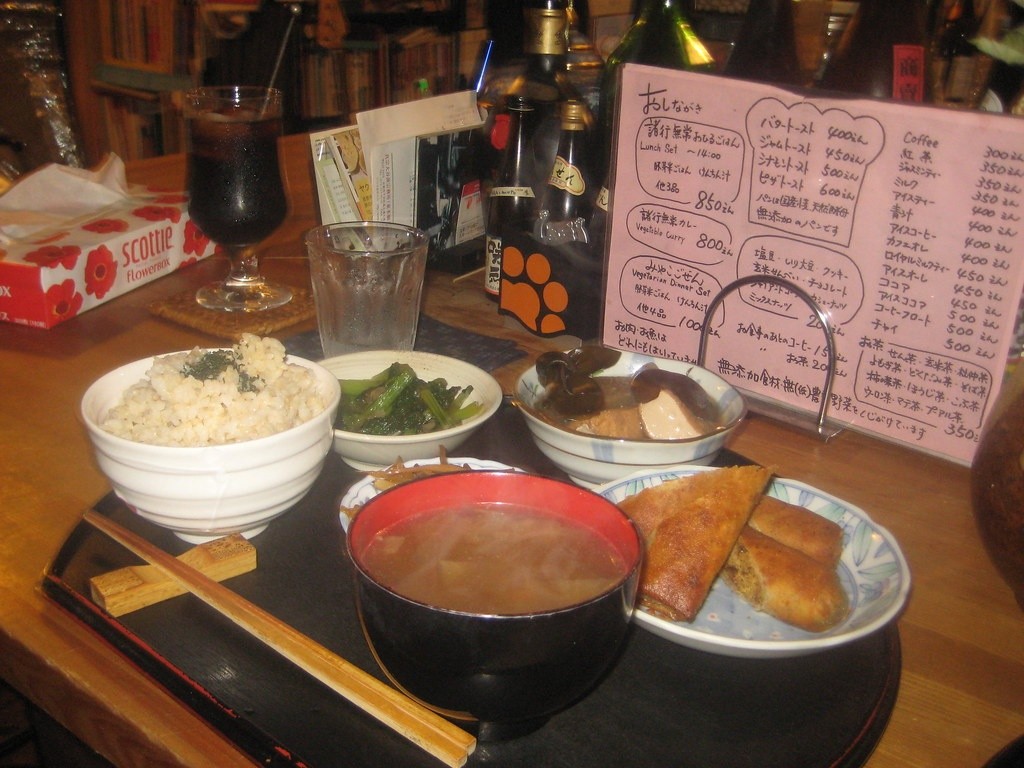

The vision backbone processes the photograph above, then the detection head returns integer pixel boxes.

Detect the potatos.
[341,445,472,516]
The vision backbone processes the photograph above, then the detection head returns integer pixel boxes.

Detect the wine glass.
[181,83,294,312]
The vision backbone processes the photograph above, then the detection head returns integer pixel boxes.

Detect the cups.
[306,222,429,359]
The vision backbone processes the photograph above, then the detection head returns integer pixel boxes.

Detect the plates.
[588,465,910,658]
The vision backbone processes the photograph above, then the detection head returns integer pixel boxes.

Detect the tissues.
[0,149,222,329]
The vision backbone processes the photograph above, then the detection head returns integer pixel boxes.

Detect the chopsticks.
[83,507,477,768]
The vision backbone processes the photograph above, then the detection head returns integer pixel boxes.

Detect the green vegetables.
[333,361,480,436]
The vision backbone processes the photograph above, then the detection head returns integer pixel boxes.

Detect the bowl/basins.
[346,469,642,743]
[80,348,342,545]
[316,350,502,471]
[514,349,750,491]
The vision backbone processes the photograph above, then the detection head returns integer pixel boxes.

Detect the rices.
[98,334,325,446]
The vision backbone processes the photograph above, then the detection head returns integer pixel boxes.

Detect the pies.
[610,465,848,631]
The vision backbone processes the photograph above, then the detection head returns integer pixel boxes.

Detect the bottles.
[502,0,582,127]
[530,97,597,245]
[722,0,802,87]
[588,170,610,260]
[931,0,993,110]
[817,0,936,106]
[485,93,536,302]
[588,0,716,194]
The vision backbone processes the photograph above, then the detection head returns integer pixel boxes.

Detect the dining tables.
[0,132,1022,767]
